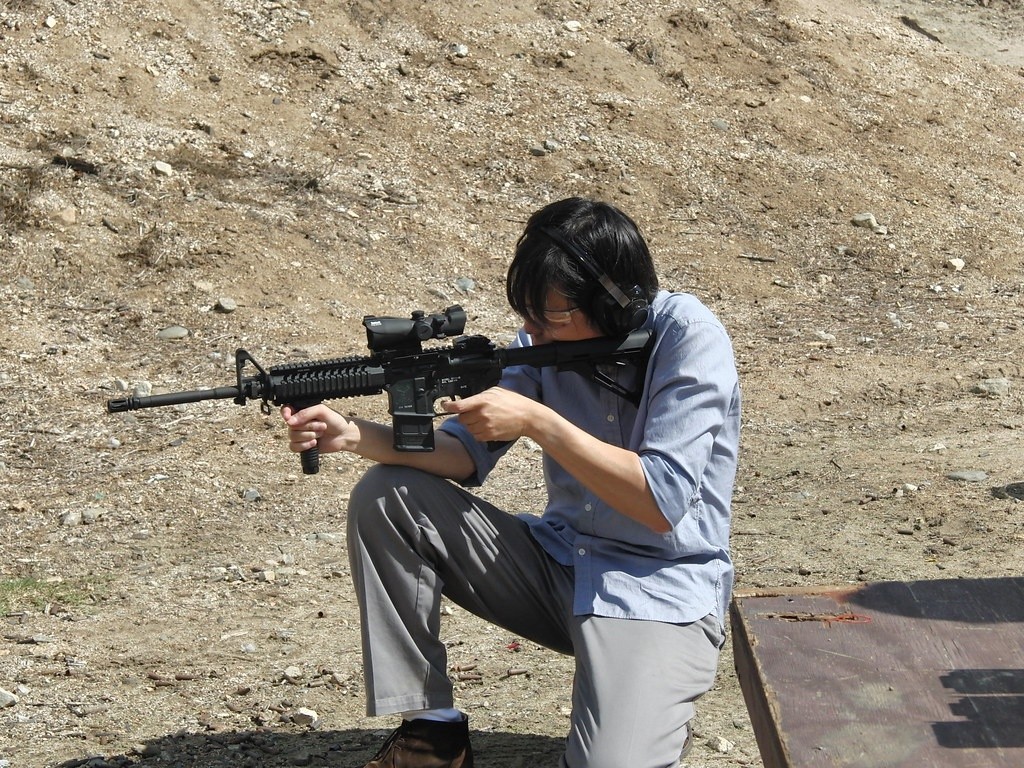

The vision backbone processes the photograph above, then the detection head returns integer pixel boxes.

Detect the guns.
[106,303,659,477]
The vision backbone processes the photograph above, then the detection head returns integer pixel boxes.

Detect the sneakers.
[363,712,474,768]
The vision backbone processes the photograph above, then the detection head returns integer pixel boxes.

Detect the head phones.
[541,227,649,336]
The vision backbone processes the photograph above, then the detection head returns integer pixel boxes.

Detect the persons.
[281,197,741,768]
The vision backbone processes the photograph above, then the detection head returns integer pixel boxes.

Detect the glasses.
[517,306,581,327]
